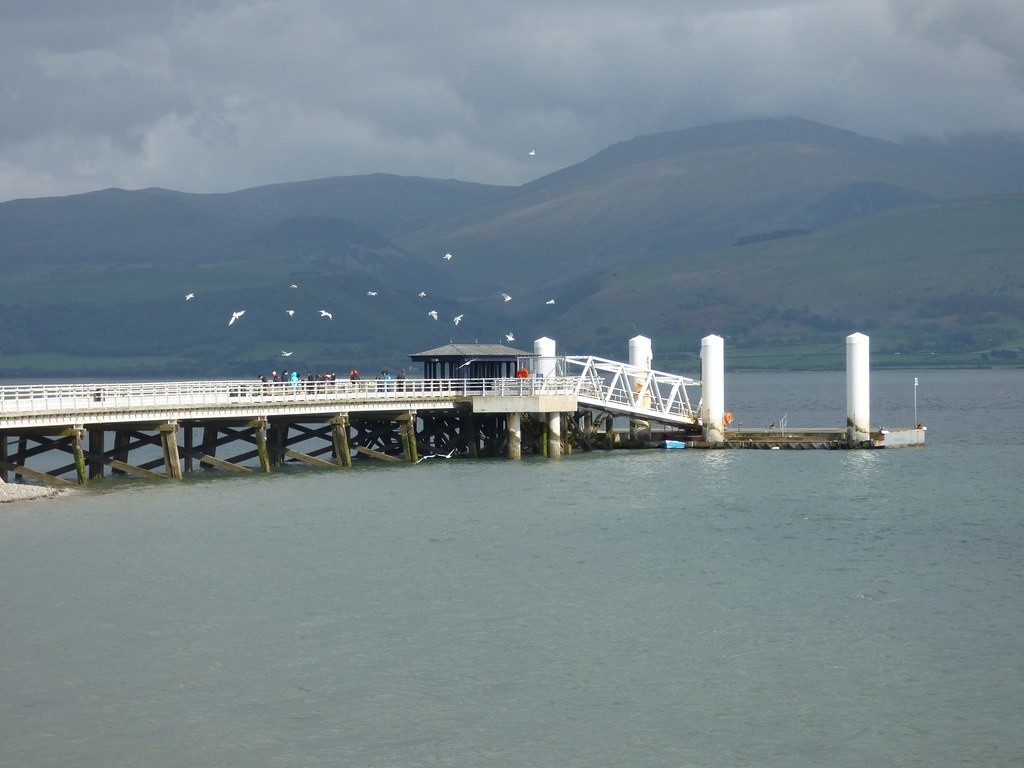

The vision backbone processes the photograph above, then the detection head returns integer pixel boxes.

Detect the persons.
[395,368,408,392]
[258,369,392,393]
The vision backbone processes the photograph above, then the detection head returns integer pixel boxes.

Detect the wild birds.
[505,332,515,342]
[317,310,333,320]
[429,310,439,320]
[286,310,295,317]
[453,314,464,326]
[228,310,246,325]
[545,299,556,305]
[443,253,452,260]
[418,291,427,298]
[185,292,195,301]
[502,293,512,302]
[281,350,294,357]
[459,358,478,368]
[366,291,378,297]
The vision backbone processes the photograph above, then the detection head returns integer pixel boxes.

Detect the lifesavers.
[517,369,529,378]
[726,413,732,424]
